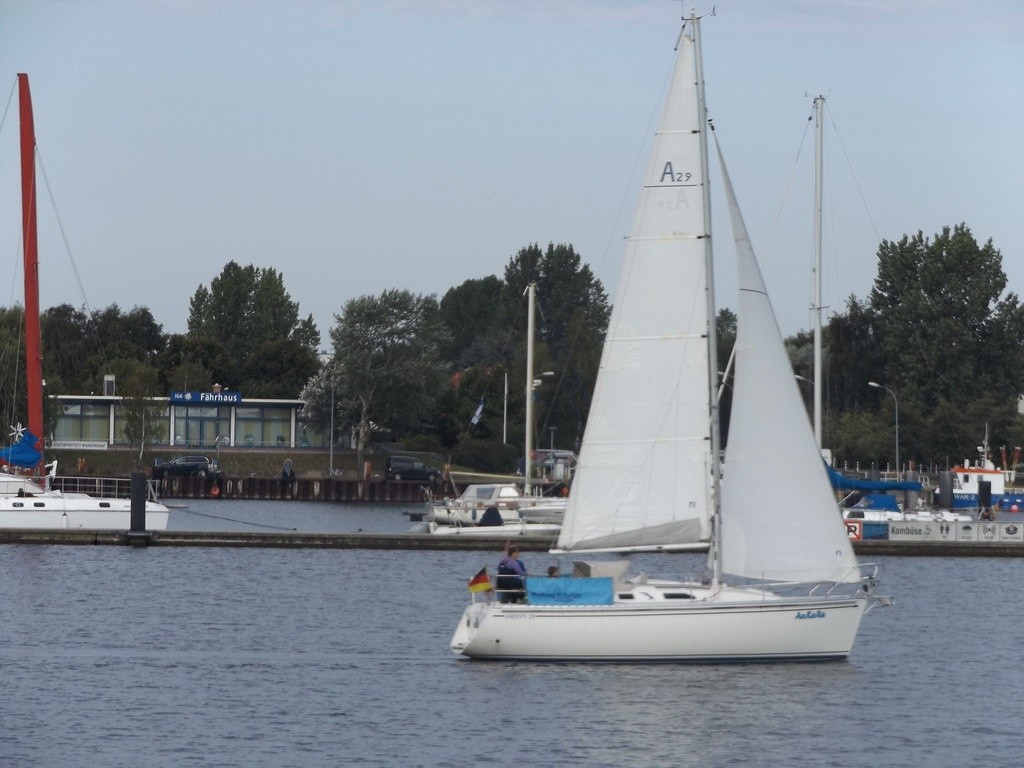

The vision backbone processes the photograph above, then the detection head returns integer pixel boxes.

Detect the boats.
[825,421,1024,557]
[401,279,577,538]
[0,71,172,532]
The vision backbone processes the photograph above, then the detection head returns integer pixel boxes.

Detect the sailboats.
[446,5,894,667]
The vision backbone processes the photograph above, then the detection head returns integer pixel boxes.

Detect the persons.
[548,566,559,577]
[176,433,181,445]
[274,458,294,498]
[223,435,229,446]
[496,546,527,604]
[246,434,255,448]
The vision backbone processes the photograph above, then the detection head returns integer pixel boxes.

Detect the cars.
[384,455,441,482]
[152,453,216,478]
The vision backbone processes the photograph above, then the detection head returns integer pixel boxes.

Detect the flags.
[468,567,491,592]
[471,399,484,424]
[0,419,42,468]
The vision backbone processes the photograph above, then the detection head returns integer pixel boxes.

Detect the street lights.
[868,381,899,479]
[525,370,556,499]
